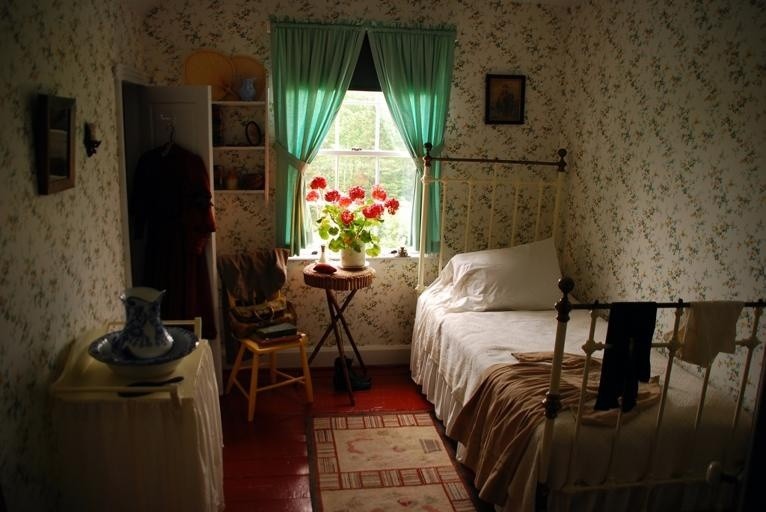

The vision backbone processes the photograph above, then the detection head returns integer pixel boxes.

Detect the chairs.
[217,248,315,421]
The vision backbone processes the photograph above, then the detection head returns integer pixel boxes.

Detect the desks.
[293,260,376,406]
[45,317,225,512]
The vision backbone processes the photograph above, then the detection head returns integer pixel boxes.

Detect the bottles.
[212,105,222,147]
[318,244,327,264]
[213,163,238,190]
[239,79,257,102]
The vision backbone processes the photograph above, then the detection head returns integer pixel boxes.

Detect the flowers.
[307,177,399,258]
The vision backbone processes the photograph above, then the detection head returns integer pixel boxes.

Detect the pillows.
[429,236,582,312]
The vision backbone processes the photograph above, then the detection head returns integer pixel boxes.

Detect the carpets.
[305,408,497,512]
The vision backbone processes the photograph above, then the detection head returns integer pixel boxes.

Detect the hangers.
[147,125,176,155]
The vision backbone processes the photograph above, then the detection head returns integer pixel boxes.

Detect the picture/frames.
[483,75,525,125]
[37,92,75,195]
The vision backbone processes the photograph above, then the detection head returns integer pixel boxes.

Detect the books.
[248,322,301,348]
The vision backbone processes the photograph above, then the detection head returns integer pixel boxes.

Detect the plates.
[245,121,263,146]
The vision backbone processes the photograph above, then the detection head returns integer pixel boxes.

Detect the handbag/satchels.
[228,295,301,346]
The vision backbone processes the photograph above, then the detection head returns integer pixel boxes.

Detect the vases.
[340,238,365,269]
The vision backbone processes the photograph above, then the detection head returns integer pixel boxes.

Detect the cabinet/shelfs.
[212,72,269,209]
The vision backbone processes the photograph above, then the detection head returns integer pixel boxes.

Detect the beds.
[408,143,766,511]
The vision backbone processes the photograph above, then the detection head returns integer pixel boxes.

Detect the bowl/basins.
[85,325,202,378]
[243,173,263,189]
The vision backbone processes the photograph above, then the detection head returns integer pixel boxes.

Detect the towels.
[671,300,744,369]
[592,302,657,415]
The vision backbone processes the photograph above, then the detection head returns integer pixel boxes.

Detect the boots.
[333,357,371,391]
